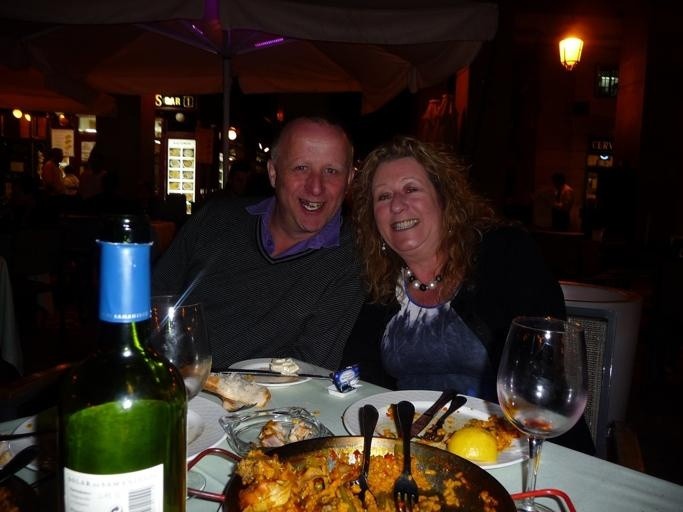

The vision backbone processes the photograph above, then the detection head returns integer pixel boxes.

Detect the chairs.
[551,306,620,458]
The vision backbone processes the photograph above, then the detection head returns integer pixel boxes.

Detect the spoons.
[346,403,380,506]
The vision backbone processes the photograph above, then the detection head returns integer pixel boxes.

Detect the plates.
[228,357,314,387]
[344,389,536,469]
[9,395,230,472]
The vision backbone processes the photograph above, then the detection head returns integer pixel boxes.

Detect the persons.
[224,158,256,197]
[42,149,63,198]
[350,136,600,457]
[147,116,371,370]
[542,170,576,232]
[8,174,60,231]
[83,171,143,215]
[64,164,80,195]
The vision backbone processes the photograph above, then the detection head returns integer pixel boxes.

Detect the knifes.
[401,386,457,440]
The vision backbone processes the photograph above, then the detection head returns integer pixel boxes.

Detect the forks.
[415,396,467,442]
[393,401,419,507]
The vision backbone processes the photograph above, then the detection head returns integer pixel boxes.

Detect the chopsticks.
[214,365,331,379]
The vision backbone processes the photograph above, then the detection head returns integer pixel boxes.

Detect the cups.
[152,295,213,408]
[497,318,588,508]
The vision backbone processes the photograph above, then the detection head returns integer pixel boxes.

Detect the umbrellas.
[0,0,515,189]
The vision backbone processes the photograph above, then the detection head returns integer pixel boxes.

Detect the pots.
[187,436,575,507]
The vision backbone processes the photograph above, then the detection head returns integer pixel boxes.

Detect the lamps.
[591,55,621,97]
[557,33,584,72]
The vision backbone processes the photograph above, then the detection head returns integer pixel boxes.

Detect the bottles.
[587,141,612,171]
[58,212,186,512]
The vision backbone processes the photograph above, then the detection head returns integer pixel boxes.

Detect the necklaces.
[397,257,452,292]
